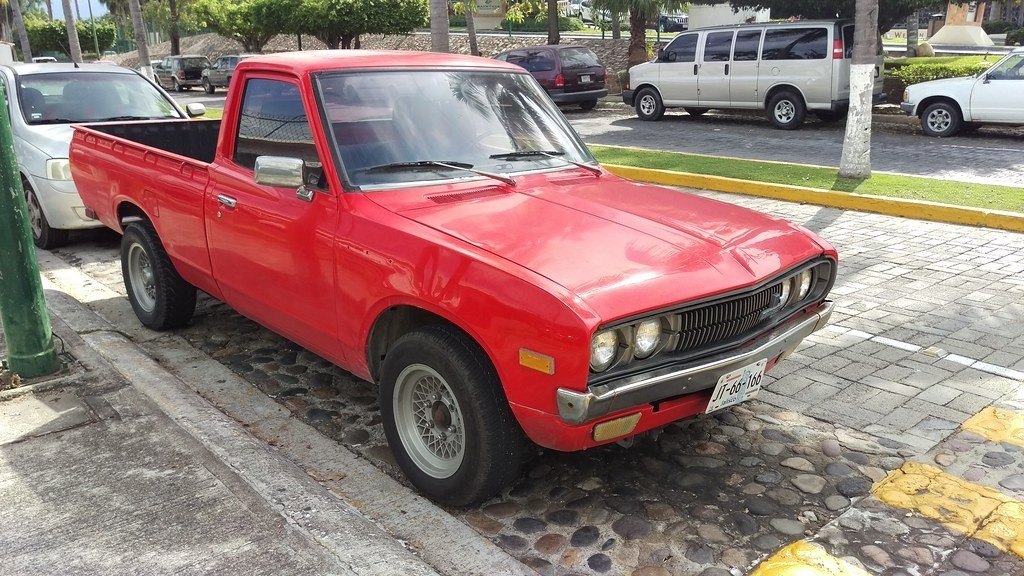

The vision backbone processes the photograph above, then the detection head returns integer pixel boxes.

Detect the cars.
[200,55,254,94]
[899,44,1024,137]
[31,56,58,63]
[488,45,609,110]
[152,54,213,92]
[0,60,207,250]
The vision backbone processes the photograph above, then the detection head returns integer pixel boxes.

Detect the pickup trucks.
[67,52,841,513]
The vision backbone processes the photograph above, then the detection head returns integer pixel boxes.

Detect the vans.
[621,18,884,131]
